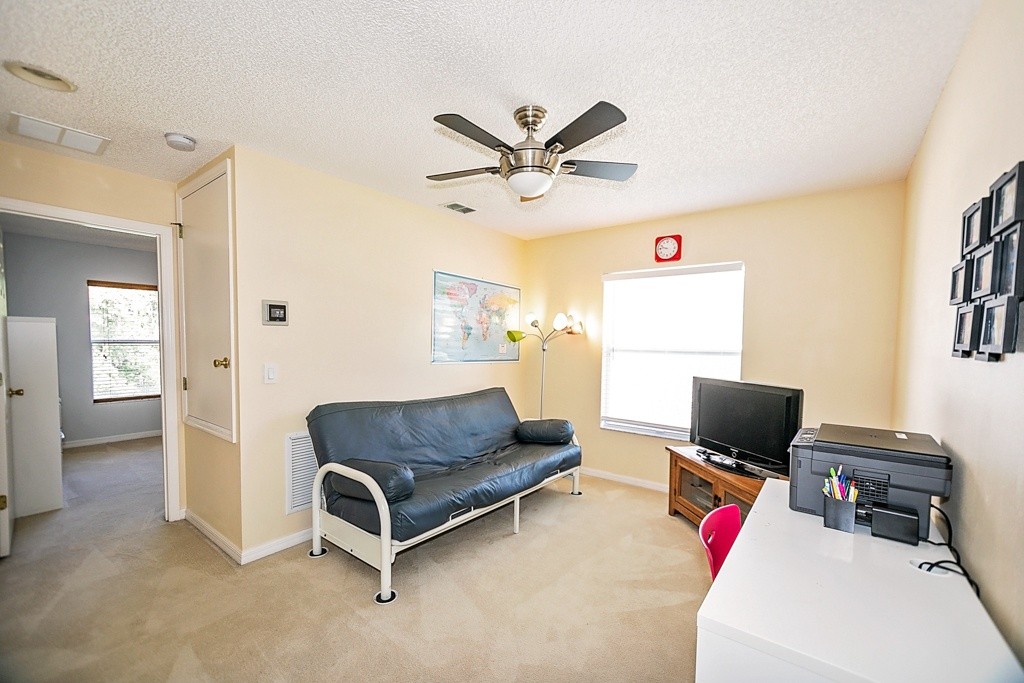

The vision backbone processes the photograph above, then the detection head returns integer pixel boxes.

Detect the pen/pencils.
[822,464,858,502]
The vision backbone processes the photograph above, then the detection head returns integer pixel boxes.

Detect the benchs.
[302,386,583,604]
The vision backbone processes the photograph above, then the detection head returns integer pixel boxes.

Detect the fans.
[424,100,639,204]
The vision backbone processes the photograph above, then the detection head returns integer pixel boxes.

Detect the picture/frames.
[949,160,1024,362]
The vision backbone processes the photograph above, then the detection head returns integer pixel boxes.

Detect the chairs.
[698,504,742,583]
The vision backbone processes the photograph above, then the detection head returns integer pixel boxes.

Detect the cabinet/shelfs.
[5,316,66,518]
[664,445,789,530]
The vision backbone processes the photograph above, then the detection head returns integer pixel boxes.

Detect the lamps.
[505,167,556,199]
[164,132,196,153]
[506,310,585,420]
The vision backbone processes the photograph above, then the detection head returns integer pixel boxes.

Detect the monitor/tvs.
[689,377,803,477]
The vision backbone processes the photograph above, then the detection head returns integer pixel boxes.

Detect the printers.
[789,423,954,542]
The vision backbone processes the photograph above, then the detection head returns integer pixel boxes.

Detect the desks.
[696,476,1024,683]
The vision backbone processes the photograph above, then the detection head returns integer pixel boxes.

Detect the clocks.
[655,234,682,263]
